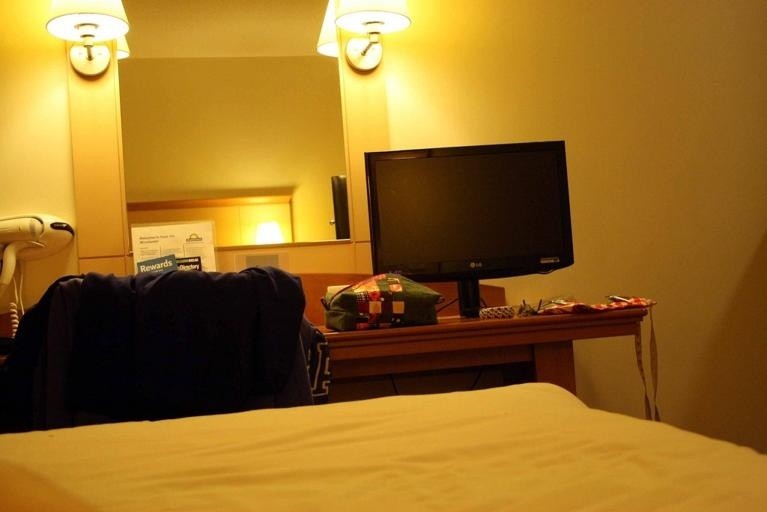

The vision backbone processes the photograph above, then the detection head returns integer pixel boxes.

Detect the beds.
[0,378,767,510]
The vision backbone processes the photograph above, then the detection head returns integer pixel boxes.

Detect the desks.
[306,307,648,397]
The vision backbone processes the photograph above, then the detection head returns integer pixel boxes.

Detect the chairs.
[2,272,316,433]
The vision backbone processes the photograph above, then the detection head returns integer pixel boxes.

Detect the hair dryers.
[0,216,43,286]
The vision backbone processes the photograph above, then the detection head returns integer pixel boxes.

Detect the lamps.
[334,2,414,74]
[41,0,132,80]
[117,35,130,62]
[315,2,336,58]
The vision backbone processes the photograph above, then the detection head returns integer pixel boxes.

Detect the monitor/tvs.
[363,140,574,316]
[331,175,349,239]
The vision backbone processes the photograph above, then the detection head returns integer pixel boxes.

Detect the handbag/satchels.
[320,272,442,331]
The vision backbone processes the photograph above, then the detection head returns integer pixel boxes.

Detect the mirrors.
[117,0,352,255]
